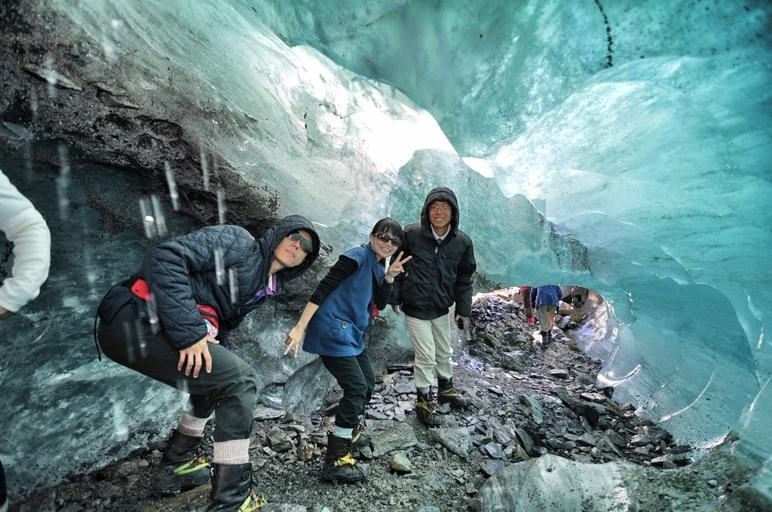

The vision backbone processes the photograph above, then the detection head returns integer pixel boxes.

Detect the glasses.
[289,231,313,253]
[378,233,403,246]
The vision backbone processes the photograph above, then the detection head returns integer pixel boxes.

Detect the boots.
[417,386,441,424]
[439,377,469,408]
[206,463,260,512]
[154,429,209,493]
[322,413,369,482]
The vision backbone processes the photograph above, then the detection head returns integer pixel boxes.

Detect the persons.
[282,217,412,483]
[1,170,51,511]
[389,187,476,425]
[93,214,320,512]
[518,285,589,347]
